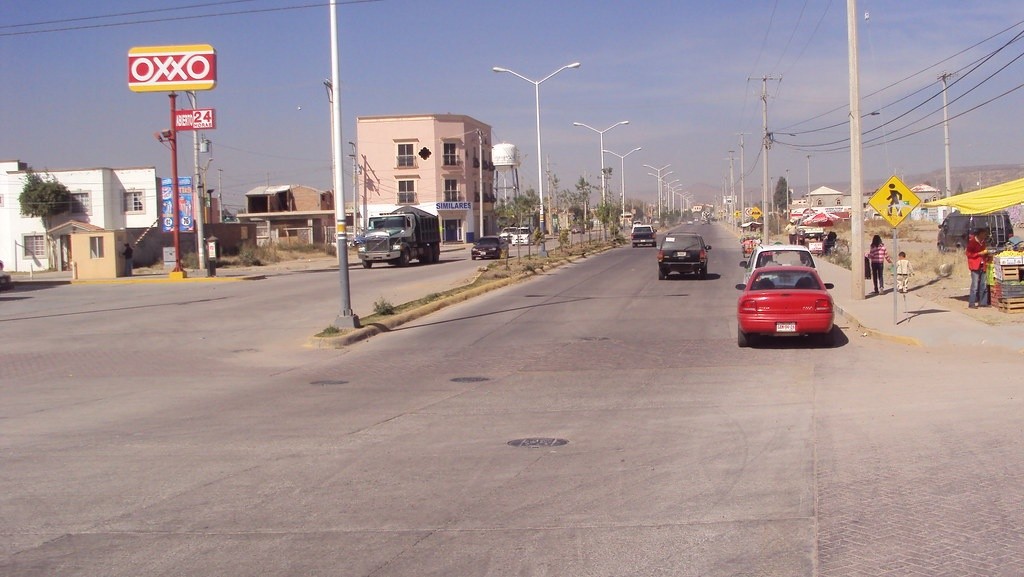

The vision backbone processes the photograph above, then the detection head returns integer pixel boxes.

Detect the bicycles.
[835,239,849,253]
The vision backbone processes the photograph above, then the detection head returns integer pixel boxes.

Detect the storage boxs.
[984,255,1024,314]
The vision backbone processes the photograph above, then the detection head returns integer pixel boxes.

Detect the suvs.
[656,233,711,280]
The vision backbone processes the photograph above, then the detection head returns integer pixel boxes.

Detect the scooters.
[740,235,762,258]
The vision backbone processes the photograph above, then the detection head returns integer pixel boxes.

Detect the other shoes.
[899,289,902,293]
[972,306,977,309]
[880,288,883,294]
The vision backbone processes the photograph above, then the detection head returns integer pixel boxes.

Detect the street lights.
[663,180,687,217]
[493,63,581,257]
[574,121,629,241]
[648,171,673,205]
[643,164,671,218]
[603,147,642,229]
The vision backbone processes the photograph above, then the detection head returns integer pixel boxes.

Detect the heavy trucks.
[354,206,442,269]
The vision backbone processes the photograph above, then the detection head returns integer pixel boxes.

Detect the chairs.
[761,254,773,265]
[646,229,651,232]
[757,277,775,289]
[795,277,814,288]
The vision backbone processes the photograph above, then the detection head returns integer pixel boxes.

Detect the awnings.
[921,177,1024,216]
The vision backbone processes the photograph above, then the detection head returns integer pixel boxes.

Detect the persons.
[122,243,133,276]
[865,235,893,294]
[966,229,989,309]
[785,218,798,245]
[893,252,915,293]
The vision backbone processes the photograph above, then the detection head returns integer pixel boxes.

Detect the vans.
[937,209,1014,253]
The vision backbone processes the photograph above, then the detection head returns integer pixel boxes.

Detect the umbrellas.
[803,210,840,223]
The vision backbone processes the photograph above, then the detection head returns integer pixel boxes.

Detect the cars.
[735,266,834,347]
[500,226,517,242]
[471,236,509,260]
[738,244,818,286]
[633,221,641,226]
[631,226,658,247]
[687,220,693,225]
[510,227,534,246]
